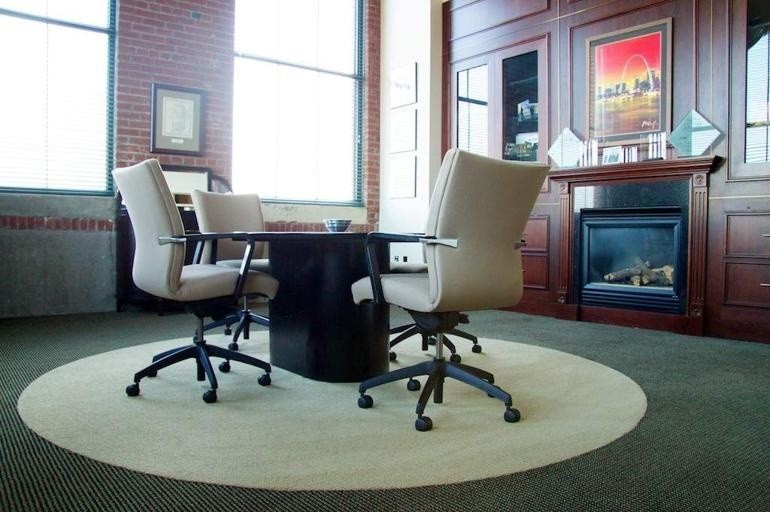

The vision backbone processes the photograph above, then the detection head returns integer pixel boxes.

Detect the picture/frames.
[151,83,208,158]
[583,15,674,150]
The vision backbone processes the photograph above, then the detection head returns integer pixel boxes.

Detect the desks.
[231,230,428,384]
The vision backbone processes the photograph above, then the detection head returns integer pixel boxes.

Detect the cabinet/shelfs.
[444,1,770,351]
[118,205,218,316]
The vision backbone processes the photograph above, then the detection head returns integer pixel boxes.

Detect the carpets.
[17,331,647,493]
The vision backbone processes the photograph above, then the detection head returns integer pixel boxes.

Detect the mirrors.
[118,164,214,207]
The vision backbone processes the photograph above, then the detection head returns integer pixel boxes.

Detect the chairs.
[189,187,270,352]
[112,159,279,403]
[351,147,551,434]
[386,233,482,363]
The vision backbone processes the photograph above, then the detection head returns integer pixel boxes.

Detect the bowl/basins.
[323,219,352,232]
